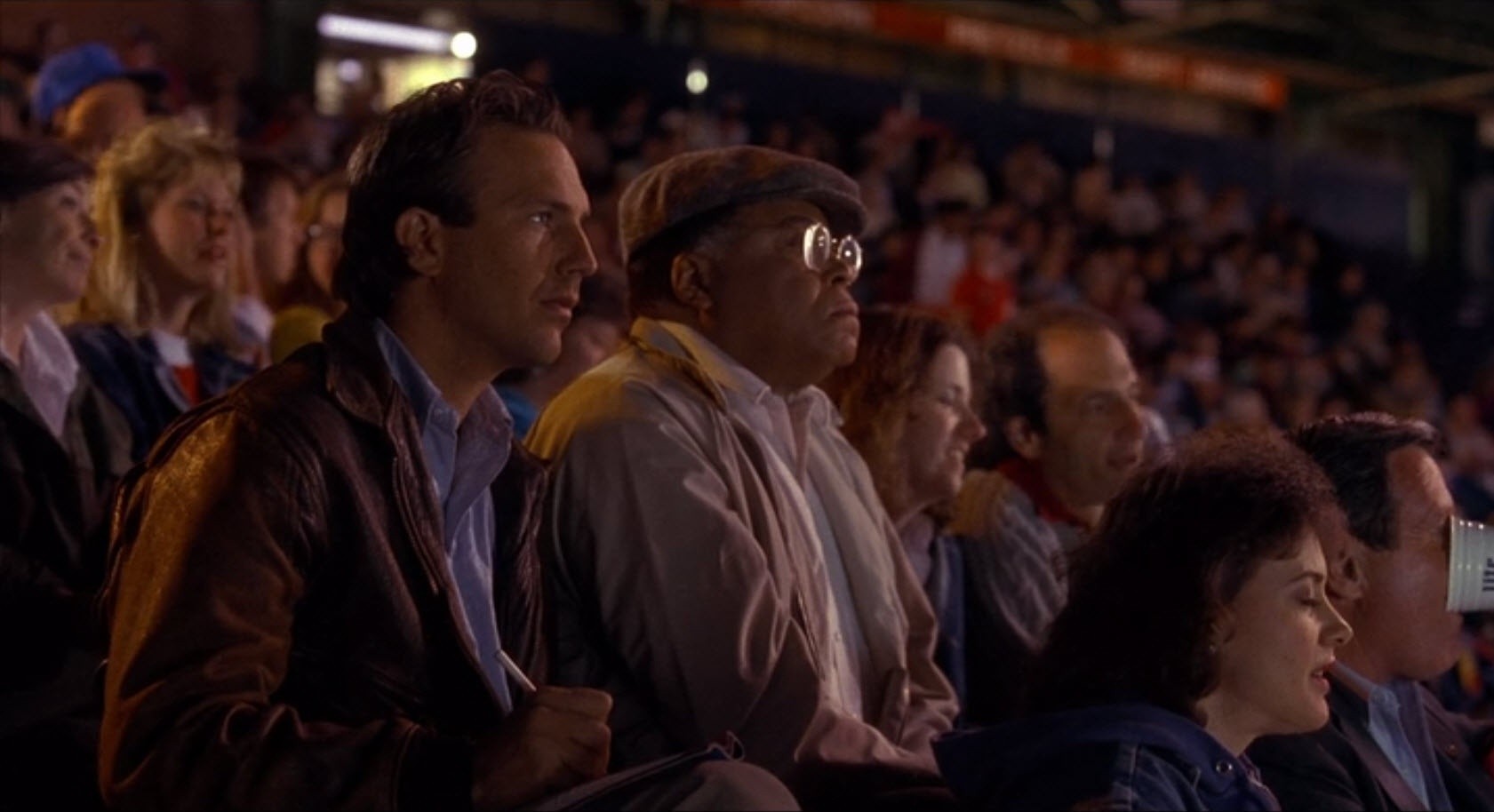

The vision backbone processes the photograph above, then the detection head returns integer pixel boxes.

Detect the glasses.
[720,223,863,282]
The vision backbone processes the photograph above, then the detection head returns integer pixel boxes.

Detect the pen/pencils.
[492,647,534,689]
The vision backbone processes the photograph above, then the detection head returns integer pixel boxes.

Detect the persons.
[0,30,1493,812]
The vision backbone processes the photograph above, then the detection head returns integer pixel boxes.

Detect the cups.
[1445,514,1494,612]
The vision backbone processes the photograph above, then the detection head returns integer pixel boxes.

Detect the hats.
[617,146,864,270]
[33,46,169,133]
